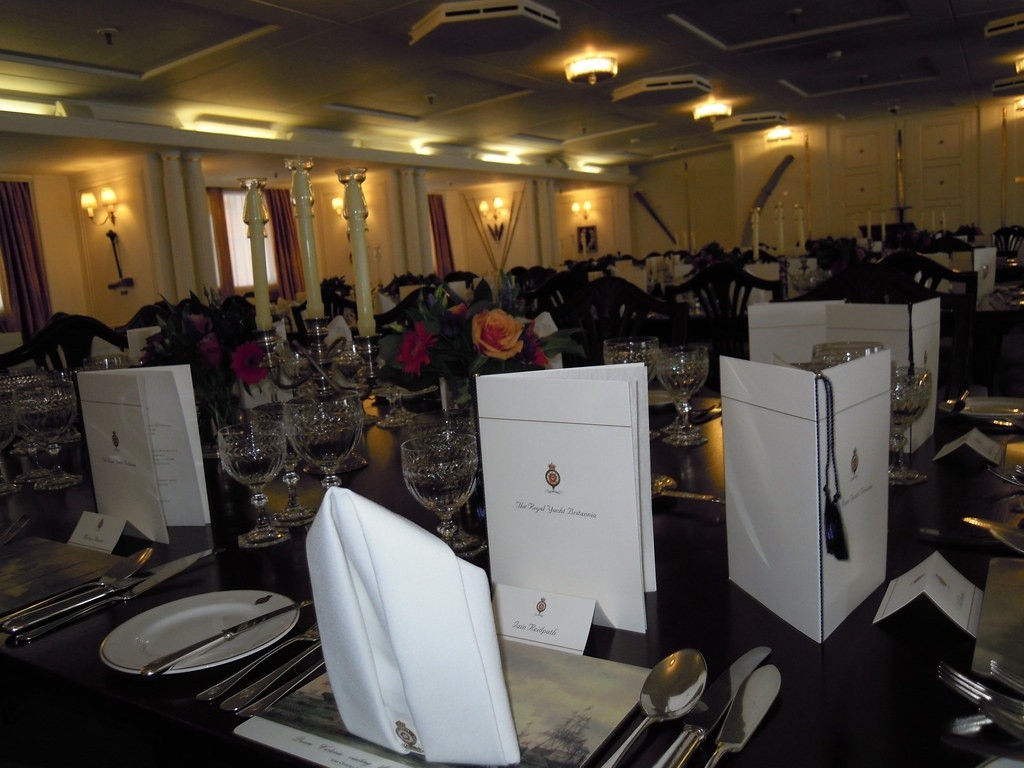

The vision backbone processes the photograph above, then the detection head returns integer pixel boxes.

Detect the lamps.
[81,187,116,225]
[564,52,619,85]
[693,104,732,125]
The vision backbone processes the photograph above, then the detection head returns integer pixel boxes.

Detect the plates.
[937,397,1024,420]
[99,588,300,676]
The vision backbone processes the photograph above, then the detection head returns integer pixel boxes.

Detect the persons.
[323,278,356,321]
[879,224,986,255]
[840,248,877,295]
[673,242,778,320]
[805,236,857,269]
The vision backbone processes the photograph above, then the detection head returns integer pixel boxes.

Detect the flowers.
[375,284,588,405]
[141,286,270,426]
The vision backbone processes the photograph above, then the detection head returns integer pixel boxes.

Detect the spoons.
[0,548,154,625]
[594,649,708,768]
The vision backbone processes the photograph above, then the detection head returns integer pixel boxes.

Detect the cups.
[812,341,883,374]
[82,353,126,372]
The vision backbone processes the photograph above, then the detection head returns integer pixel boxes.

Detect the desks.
[0,395,1024,768]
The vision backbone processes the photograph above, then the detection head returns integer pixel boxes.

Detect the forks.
[0,514,31,546]
[934,658,1024,740]
[196,621,321,701]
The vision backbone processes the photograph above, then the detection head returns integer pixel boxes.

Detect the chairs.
[0,305,162,373]
[379,220,1024,394]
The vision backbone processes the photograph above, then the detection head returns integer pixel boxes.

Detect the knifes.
[2,549,214,634]
[949,390,970,415]
[16,552,202,641]
[966,515,1024,539]
[140,599,313,677]
[653,645,771,768]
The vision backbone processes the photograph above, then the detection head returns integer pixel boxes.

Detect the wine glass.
[0,366,84,495]
[601,336,711,444]
[213,348,488,558]
[889,368,932,487]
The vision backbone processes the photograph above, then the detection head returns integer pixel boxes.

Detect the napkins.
[304,486,520,766]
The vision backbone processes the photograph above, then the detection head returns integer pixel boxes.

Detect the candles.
[285,157,323,318]
[243,178,272,331]
[753,203,805,260]
[867,210,886,242]
[337,167,376,337]
[921,211,947,230]
